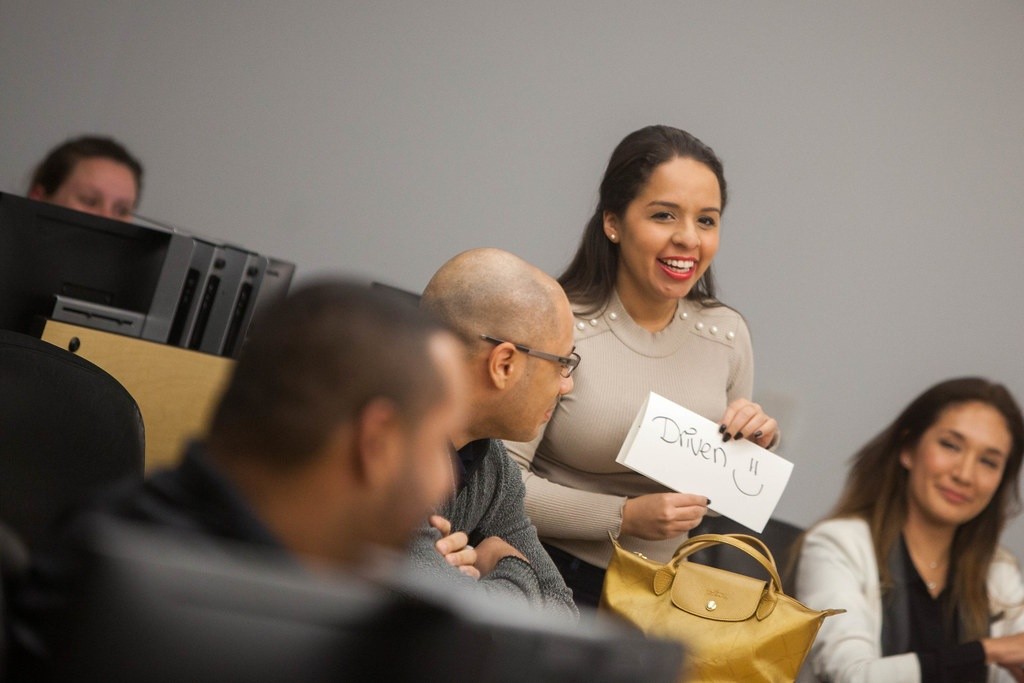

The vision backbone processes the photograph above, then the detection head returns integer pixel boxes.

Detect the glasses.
[481,334,582,378]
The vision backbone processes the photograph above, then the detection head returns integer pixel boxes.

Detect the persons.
[785,376,1024,683]
[0,278,478,683]
[501,124,782,622]
[24,134,145,223]
[421,246,582,635]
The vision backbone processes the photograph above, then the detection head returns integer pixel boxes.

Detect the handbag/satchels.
[598,528,847,683]
